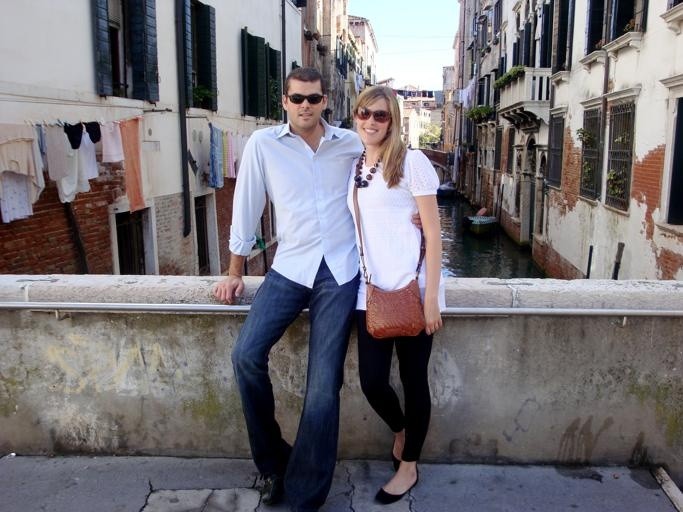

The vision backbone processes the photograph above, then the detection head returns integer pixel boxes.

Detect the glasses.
[356,106,392,123]
[287,93,323,104]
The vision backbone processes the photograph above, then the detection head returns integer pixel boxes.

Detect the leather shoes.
[392,426,406,471]
[375,463,419,505]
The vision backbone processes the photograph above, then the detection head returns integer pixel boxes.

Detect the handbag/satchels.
[365,279,425,338]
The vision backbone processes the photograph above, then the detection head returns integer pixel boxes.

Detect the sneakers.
[261,440,294,504]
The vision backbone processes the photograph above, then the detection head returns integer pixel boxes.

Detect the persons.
[342,87,443,504]
[212,68,421,511]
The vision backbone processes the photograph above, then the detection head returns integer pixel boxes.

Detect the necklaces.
[355,148,383,188]
